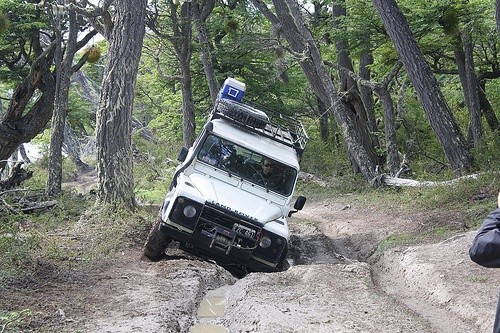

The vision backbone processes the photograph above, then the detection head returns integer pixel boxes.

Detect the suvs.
[143,91,310,273]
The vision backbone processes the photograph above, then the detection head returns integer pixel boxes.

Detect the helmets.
[209,142,237,167]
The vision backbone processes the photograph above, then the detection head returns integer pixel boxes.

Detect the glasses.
[264,163,274,168]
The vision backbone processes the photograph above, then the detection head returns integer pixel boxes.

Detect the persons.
[469,187,500,333]
[251,157,285,194]
